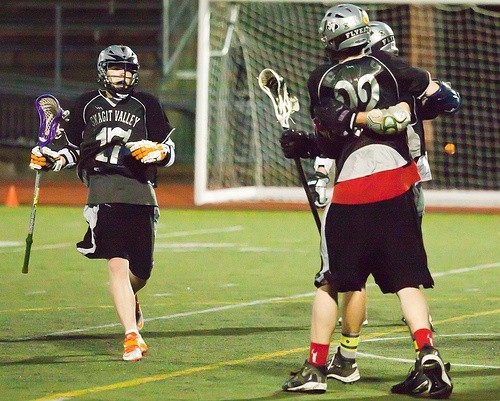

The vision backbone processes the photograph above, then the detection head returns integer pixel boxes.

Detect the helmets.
[368,20,400,57]
[319,3,371,51]
[96,44,141,97]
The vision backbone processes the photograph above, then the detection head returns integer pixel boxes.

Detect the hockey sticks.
[258,68,321,238]
[21,93,69,274]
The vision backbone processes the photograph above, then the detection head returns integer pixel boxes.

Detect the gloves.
[28,146,63,172]
[280,129,316,161]
[314,98,358,132]
[307,171,330,208]
[125,138,170,165]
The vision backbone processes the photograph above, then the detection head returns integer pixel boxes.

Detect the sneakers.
[122,329,148,363]
[418,343,454,398]
[390,359,451,394]
[134,294,144,330]
[282,359,328,391]
[326,345,361,385]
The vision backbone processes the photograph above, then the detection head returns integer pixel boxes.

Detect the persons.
[29,45,176,362]
[280,4,461,399]
